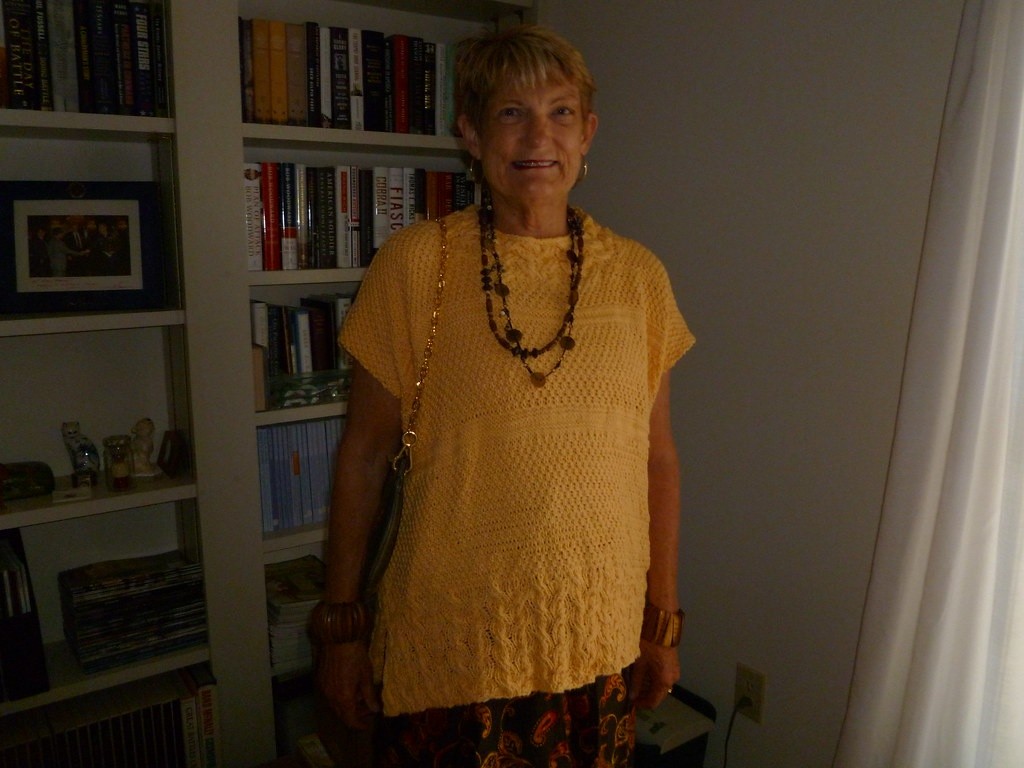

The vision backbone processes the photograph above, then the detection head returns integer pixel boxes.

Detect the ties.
[76,234,81,249]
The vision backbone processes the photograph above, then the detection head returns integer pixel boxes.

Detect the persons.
[308,24,697,768]
[29,218,131,276]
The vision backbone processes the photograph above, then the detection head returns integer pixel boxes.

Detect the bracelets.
[306,596,373,646]
[640,601,683,647]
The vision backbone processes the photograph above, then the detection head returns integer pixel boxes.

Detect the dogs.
[102,434,133,490]
[131,418,155,473]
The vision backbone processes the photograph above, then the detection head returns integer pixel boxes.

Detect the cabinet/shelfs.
[0,1,535,768]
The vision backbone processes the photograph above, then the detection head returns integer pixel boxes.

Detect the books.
[0,537,347,768]
[256,417,350,534]
[238,15,473,138]
[244,162,491,272]
[250,293,357,412]
[0,0,168,118]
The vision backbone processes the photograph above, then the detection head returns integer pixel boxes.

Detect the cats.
[61,421,100,487]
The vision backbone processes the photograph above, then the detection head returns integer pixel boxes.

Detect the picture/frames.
[0,179,168,316]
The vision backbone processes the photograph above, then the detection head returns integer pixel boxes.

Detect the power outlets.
[734,661,765,726]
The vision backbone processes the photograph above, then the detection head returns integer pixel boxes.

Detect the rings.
[667,689,672,693]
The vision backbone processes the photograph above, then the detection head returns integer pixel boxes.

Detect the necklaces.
[477,201,585,388]
